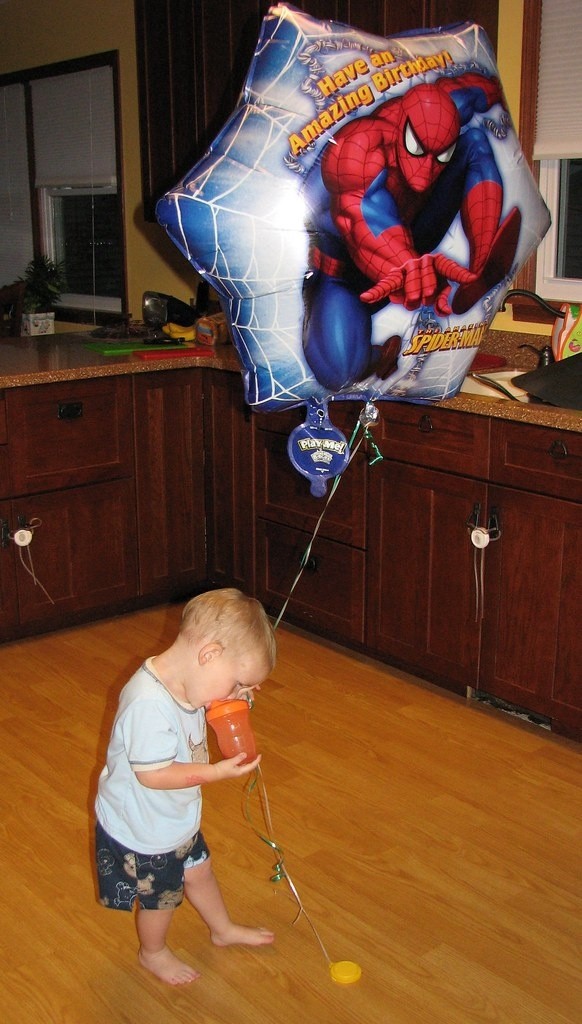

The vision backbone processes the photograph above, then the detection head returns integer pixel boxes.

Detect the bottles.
[206,700,257,765]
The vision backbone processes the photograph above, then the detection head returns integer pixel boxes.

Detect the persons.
[95,587,275,986]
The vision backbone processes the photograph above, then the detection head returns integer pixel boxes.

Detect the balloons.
[153,2,551,498]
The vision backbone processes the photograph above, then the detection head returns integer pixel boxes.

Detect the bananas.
[162,322,198,341]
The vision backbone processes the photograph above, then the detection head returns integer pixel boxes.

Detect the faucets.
[498,289,566,335]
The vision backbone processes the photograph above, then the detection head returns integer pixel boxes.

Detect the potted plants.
[14,253,72,336]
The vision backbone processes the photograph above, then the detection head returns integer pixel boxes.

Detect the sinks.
[460,367,531,404]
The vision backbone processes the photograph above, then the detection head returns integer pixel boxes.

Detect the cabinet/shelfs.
[254,400,366,645]
[133,0,499,222]
[0,375,140,640]
[365,400,581,732]
